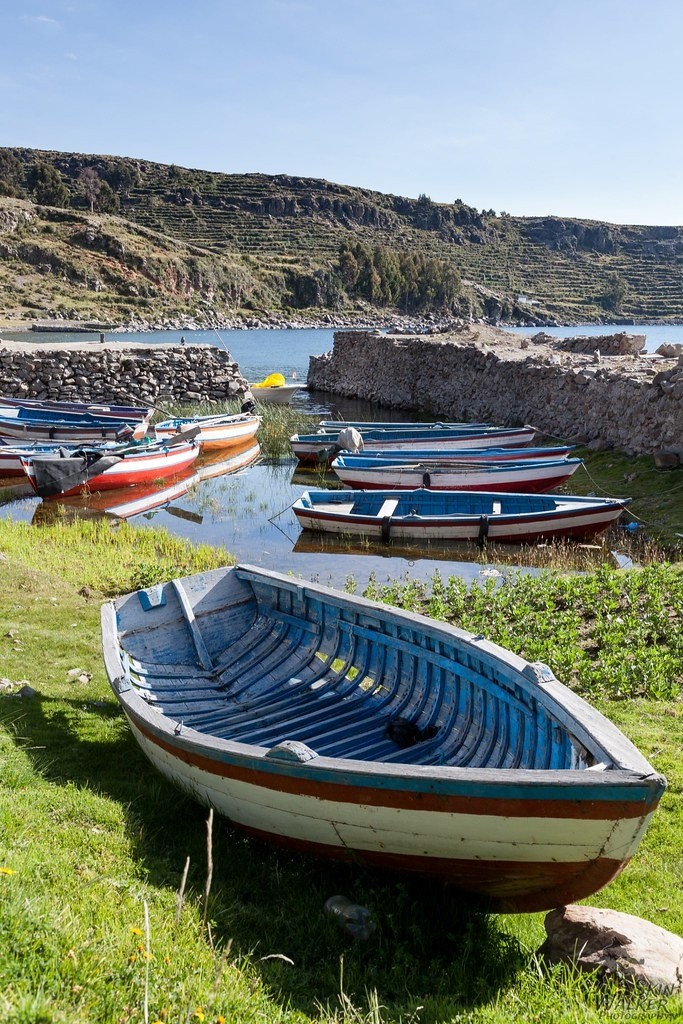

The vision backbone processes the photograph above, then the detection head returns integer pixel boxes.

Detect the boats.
[295,485,626,539]
[100,564,667,918]
[318,420,493,431]
[153,411,263,453]
[293,529,628,566]
[20,439,205,502]
[0,395,156,475]
[192,437,262,489]
[46,465,201,521]
[290,460,344,487]
[331,453,583,494]
[252,372,309,406]
[289,426,539,464]
